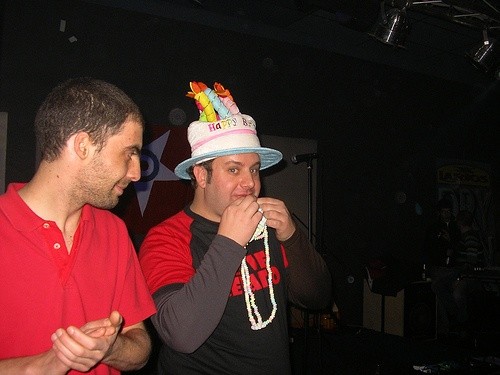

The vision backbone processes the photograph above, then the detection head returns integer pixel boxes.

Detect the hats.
[174,82,283,181]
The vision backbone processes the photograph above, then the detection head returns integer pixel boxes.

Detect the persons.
[0,76,152,375]
[137,114,336,375]
[422,198,500,365]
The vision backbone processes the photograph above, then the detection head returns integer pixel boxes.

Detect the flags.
[108,124,196,235]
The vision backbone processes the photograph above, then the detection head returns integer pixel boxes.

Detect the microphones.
[292,153,320,164]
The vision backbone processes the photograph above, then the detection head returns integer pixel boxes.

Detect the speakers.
[360,277,435,341]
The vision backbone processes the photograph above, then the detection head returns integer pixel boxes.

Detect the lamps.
[464,35,500,76]
[368,0,413,50]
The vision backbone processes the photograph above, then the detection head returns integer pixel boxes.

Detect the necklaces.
[240,223,278,330]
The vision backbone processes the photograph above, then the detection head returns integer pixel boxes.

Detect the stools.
[288,290,335,375]
